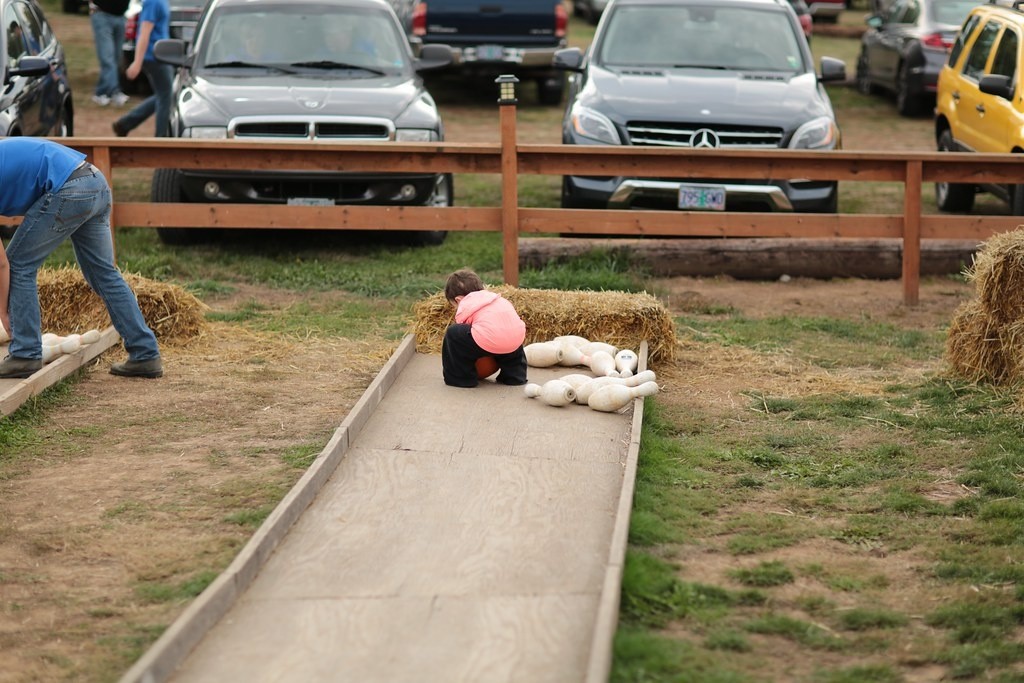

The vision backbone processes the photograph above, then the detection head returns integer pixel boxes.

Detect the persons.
[0,138,163,379]
[442,268,528,388]
[303,12,384,67]
[9,20,67,137]
[224,19,287,64]
[111,0,172,137]
[90,0,130,107]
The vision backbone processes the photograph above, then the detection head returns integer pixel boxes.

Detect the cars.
[120,0,216,95]
[856,0,1003,115]
[152,1,455,246]
[934,6,1024,211]
[411,1,573,107]
[551,0,850,247]
[1,1,76,139]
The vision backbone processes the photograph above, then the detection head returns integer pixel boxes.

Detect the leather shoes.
[110,359,163,379]
[0,356,42,379]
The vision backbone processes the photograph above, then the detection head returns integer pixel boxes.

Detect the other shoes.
[111,122,127,137]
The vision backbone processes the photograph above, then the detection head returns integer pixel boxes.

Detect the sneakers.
[92,93,131,108]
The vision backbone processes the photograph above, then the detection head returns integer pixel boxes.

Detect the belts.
[66,165,100,183]
